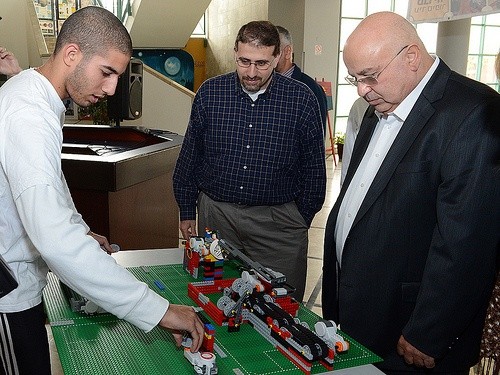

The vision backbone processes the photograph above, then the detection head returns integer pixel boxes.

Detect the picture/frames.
[62,97,78,120]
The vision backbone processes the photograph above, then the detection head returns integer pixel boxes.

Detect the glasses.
[345,44,410,87]
[236,51,277,71]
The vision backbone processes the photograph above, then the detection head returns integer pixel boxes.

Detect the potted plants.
[333,132,346,161]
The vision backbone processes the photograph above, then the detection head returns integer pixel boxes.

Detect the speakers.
[107,60,143,120]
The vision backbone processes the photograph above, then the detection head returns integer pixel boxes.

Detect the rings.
[4,47,7,51]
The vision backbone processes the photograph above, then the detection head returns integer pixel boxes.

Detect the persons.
[0,16,23,88]
[0,7,204,375]
[173,19,326,302]
[275,25,326,139]
[323,11,500,375]
